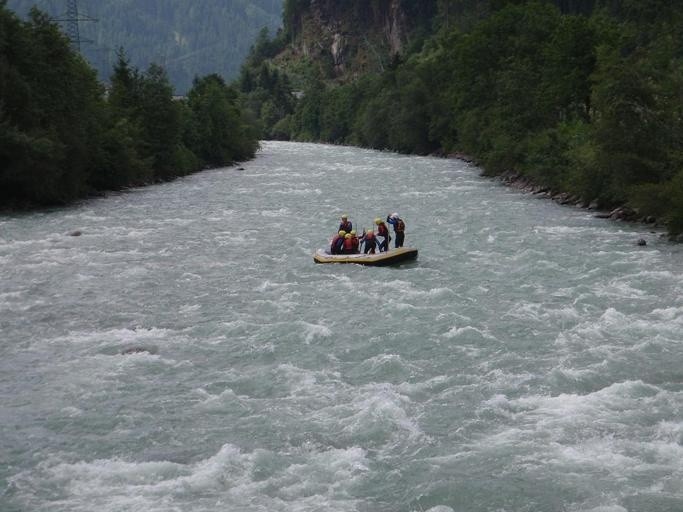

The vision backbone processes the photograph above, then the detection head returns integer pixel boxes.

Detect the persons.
[329,231,345,254]
[375,219,390,252]
[359,230,381,254]
[342,233,356,253]
[349,230,366,253]
[337,215,351,234]
[386,212,405,248]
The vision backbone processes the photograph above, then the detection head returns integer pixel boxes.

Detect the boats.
[311,245,421,266]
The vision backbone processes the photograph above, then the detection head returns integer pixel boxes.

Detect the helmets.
[351,231,356,236]
[368,230,374,235]
[339,231,346,237]
[375,218,382,225]
[392,213,399,219]
[344,234,351,239]
[341,215,347,219]
